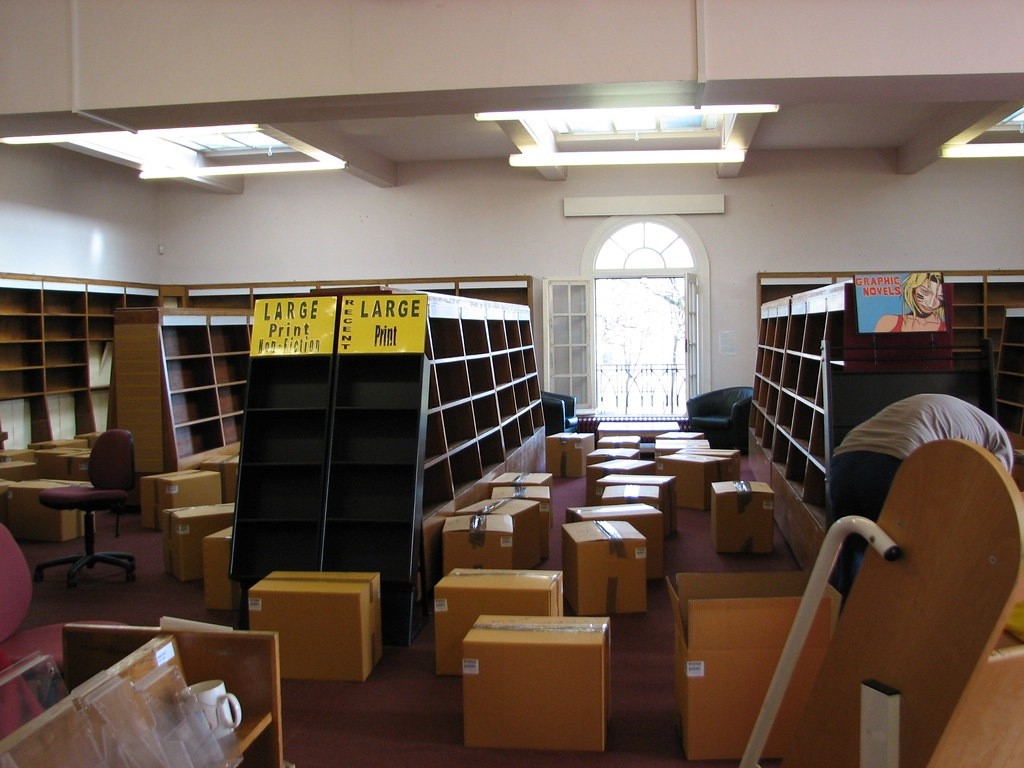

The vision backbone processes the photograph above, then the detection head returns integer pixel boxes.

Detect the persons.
[827,393,1014,623]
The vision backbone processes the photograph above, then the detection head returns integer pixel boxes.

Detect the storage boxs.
[425,434,841,752]
[249,569,381,682]
[138,454,233,612]
[1,431,103,545]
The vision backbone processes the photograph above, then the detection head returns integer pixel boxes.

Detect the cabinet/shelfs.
[0,278,1023,605]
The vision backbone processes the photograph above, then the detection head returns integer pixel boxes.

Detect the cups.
[173,680,242,740]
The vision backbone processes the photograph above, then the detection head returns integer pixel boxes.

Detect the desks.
[597,421,681,455]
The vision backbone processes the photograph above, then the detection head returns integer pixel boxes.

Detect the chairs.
[34,428,138,586]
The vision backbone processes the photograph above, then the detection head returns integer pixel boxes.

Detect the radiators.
[577,415,689,440]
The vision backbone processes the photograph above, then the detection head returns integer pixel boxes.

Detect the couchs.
[686,386,754,457]
[540,389,578,434]
